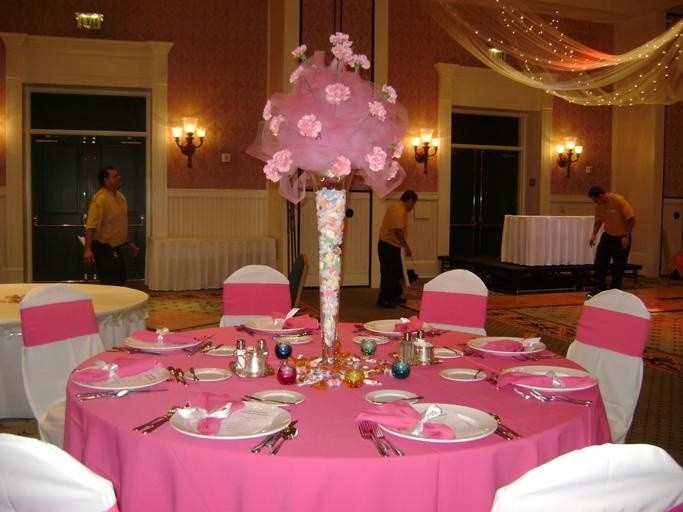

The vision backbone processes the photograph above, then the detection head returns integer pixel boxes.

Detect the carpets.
[397,287,682,466]
[0,290,339,439]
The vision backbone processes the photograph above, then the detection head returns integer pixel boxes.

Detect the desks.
[501,214,614,266]
[147,237,277,291]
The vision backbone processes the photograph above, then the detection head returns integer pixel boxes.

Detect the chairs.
[486,441,682,512]
[1,432,118,512]
[564,287,651,444]
[417,269,489,337]
[219,264,293,328]
[18,283,107,449]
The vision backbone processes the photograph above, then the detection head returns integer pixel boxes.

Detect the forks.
[511,354,565,363]
[251,420,300,457]
[184,340,212,358]
[242,395,295,407]
[133,403,191,436]
[76,389,129,401]
[167,364,187,387]
[529,388,592,406]
[357,418,404,458]
[487,412,523,441]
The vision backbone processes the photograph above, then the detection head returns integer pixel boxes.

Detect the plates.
[467,336,547,354]
[170,402,292,440]
[204,345,243,356]
[272,335,313,344]
[433,347,464,359]
[365,389,418,404]
[120,331,203,351]
[72,363,171,388]
[250,390,304,406]
[372,403,498,442]
[363,319,434,335]
[351,335,391,345]
[243,320,306,333]
[501,365,599,392]
[439,368,486,381]
[183,367,232,381]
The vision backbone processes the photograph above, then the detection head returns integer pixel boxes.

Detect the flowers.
[244,31,412,344]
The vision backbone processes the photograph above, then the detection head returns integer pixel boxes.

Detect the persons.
[588,185,635,297]
[378,190,417,307]
[82,167,140,287]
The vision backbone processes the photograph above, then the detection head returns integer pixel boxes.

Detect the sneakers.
[377,297,407,307]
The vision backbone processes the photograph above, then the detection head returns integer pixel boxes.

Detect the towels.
[70,307,319,438]
[354,312,600,440]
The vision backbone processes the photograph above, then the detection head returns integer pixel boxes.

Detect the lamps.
[410,127,441,174]
[556,137,584,179]
[171,117,206,168]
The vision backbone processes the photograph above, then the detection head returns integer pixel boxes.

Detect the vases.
[306,172,356,368]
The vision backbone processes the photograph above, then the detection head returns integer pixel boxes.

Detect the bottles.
[235,338,269,378]
[403,329,433,367]
